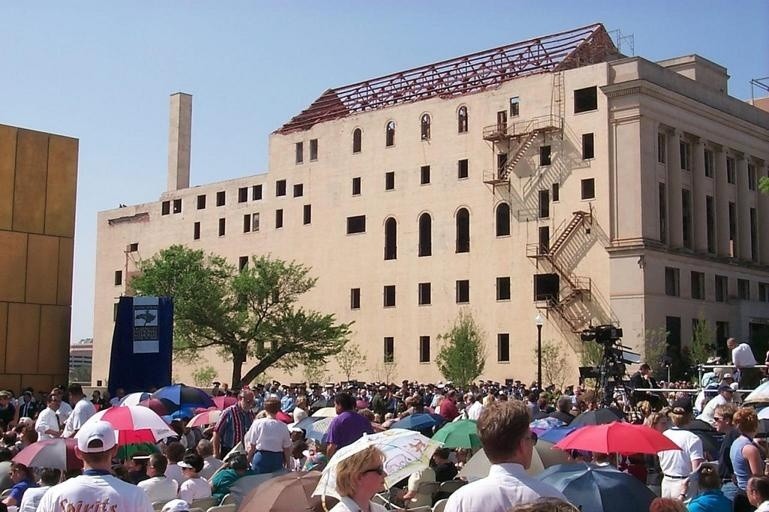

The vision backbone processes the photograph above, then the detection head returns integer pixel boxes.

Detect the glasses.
[53,398,61,402]
[526,431,538,445]
[712,416,725,421]
[362,464,385,476]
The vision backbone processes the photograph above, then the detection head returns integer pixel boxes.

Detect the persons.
[444,402,567,512]
[63,383,95,439]
[0,385,44,446]
[727,337,757,366]
[51,388,72,432]
[247,398,292,475]
[0,449,13,489]
[327,445,388,512]
[36,420,154,511]
[631,363,665,412]
[730,409,764,510]
[433,448,458,481]
[213,390,255,460]
[327,393,375,457]
[374,405,397,430]
[165,442,191,490]
[211,379,630,403]
[163,416,216,454]
[23,469,62,511]
[2,463,41,512]
[294,396,310,425]
[663,356,743,387]
[36,395,62,441]
[176,455,211,504]
[88,387,128,413]
[286,426,327,475]
[136,452,179,504]
[636,400,664,470]
[657,398,703,502]
[695,385,736,428]
[395,467,435,511]
[746,476,768,512]
[207,451,254,505]
[197,439,225,481]
[687,465,734,511]
[713,404,740,480]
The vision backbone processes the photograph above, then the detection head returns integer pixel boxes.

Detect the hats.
[241,389,254,401]
[77,420,117,453]
[176,459,192,469]
[718,383,736,392]
[670,397,694,416]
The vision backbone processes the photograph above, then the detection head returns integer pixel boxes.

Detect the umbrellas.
[567,409,618,426]
[551,421,685,469]
[538,424,588,445]
[11,439,84,472]
[186,410,221,427]
[429,420,483,449]
[135,398,180,416]
[74,405,178,465]
[152,383,217,416]
[239,472,333,512]
[391,413,442,430]
[118,392,155,404]
[311,428,445,502]
[454,439,572,481]
[534,462,657,512]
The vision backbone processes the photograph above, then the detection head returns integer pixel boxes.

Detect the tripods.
[590,345,639,425]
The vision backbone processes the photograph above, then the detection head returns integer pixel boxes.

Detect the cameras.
[581,324,623,344]
